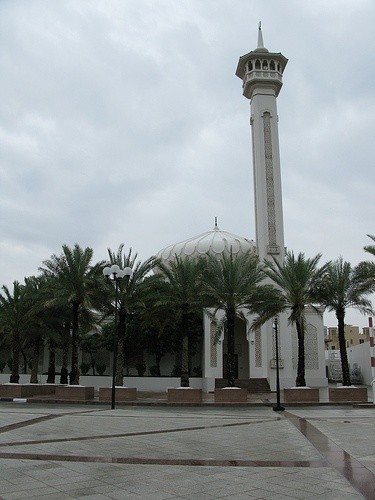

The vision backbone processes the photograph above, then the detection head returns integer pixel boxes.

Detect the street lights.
[103,265,132,411]
[273,318,285,411]
